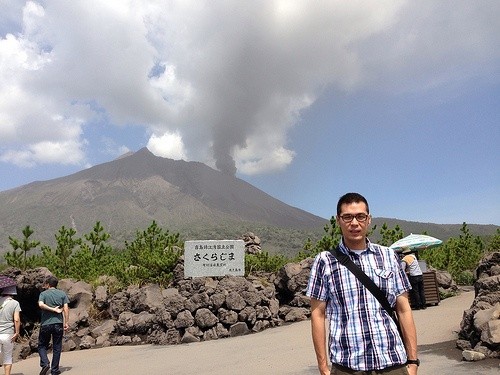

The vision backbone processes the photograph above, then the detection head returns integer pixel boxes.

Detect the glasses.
[340,212,369,222]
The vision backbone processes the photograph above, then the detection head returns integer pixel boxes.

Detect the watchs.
[406,359,420,366]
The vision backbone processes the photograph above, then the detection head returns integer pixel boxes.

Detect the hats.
[401,245,411,253]
[1,283,18,295]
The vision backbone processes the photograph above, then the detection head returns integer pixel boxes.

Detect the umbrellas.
[389,232,442,261]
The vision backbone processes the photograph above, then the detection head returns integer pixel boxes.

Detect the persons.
[38,275,70,375]
[0,275,22,375]
[306,192,420,375]
[400,246,427,310]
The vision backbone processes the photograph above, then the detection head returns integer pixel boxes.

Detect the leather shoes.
[51,369,61,375]
[40,363,50,375]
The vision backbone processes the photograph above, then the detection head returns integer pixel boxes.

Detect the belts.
[332,362,407,375]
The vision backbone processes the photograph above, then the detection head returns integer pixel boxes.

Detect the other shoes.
[415,306,426,309]
